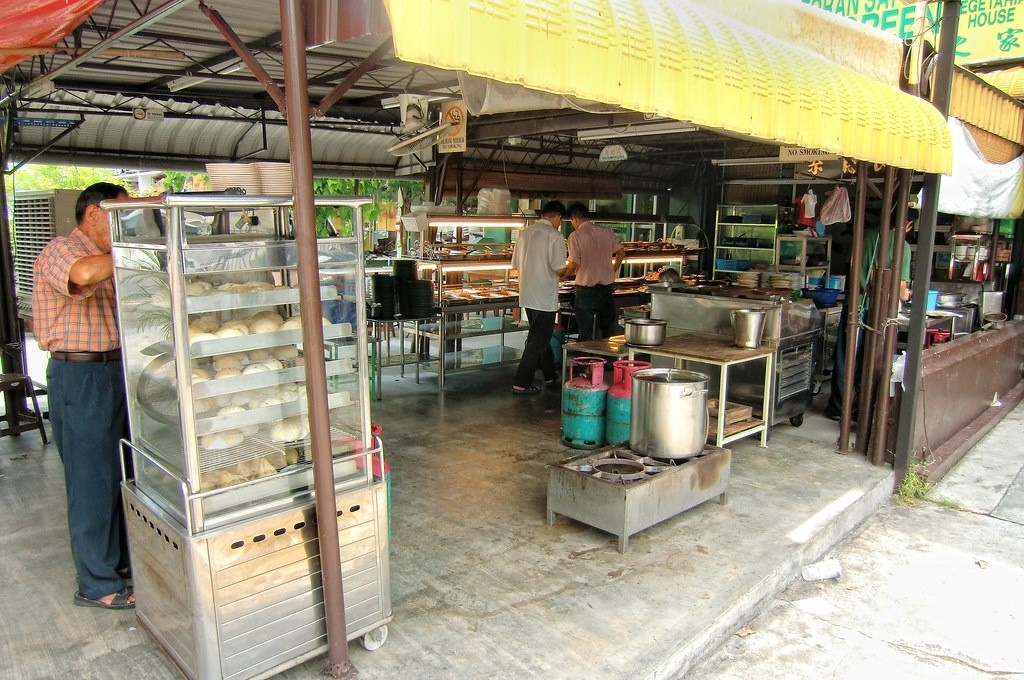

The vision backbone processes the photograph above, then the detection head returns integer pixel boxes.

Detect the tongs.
[172,188,246,197]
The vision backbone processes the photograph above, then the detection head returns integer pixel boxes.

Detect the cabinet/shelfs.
[100,195,374,517]
[399,215,709,384]
[712,204,832,289]
[906,223,1013,282]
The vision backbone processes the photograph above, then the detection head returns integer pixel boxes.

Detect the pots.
[980,311,1008,331]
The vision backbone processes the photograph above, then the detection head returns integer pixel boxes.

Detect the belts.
[578,284,611,288]
[50,349,119,361]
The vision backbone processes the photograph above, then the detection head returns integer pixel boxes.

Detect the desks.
[562,331,777,448]
[367,316,444,400]
[896,313,956,349]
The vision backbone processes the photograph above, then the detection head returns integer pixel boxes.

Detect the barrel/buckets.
[730,308,767,349]
[926,290,938,312]
[630,369,710,459]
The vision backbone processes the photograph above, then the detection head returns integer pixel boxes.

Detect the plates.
[737,271,760,288]
[206,162,293,196]
[372,260,435,319]
[770,273,793,288]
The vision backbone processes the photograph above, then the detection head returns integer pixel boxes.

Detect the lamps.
[577,121,700,141]
[167,44,261,93]
[711,157,806,166]
[380,85,463,109]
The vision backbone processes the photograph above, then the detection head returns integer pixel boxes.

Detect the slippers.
[74,585,135,609]
[544,379,554,383]
[116,562,131,579]
[511,385,542,394]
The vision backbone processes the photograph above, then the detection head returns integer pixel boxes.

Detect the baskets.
[801,288,842,305]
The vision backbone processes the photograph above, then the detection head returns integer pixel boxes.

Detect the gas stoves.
[545,439,732,554]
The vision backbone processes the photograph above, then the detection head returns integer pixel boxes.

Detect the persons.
[31,182,137,608]
[559,203,626,370]
[825,207,921,423]
[511,201,567,394]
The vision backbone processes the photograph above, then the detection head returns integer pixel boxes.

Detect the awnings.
[305,0,955,179]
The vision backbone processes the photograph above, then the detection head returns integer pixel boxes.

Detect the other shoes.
[852,411,858,421]
[824,406,841,419]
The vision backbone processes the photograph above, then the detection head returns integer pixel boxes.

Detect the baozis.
[152,280,332,449]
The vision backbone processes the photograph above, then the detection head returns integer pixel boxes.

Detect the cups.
[370,306,383,319]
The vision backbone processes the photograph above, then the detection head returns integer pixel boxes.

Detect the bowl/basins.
[801,287,841,306]
[955,244,988,261]
[624,318,668,347]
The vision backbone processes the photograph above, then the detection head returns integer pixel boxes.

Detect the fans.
[386,80,451,156]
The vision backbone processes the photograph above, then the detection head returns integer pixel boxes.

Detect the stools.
[0,373,50,446]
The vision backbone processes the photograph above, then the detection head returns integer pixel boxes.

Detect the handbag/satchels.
[819,187,852,225]
